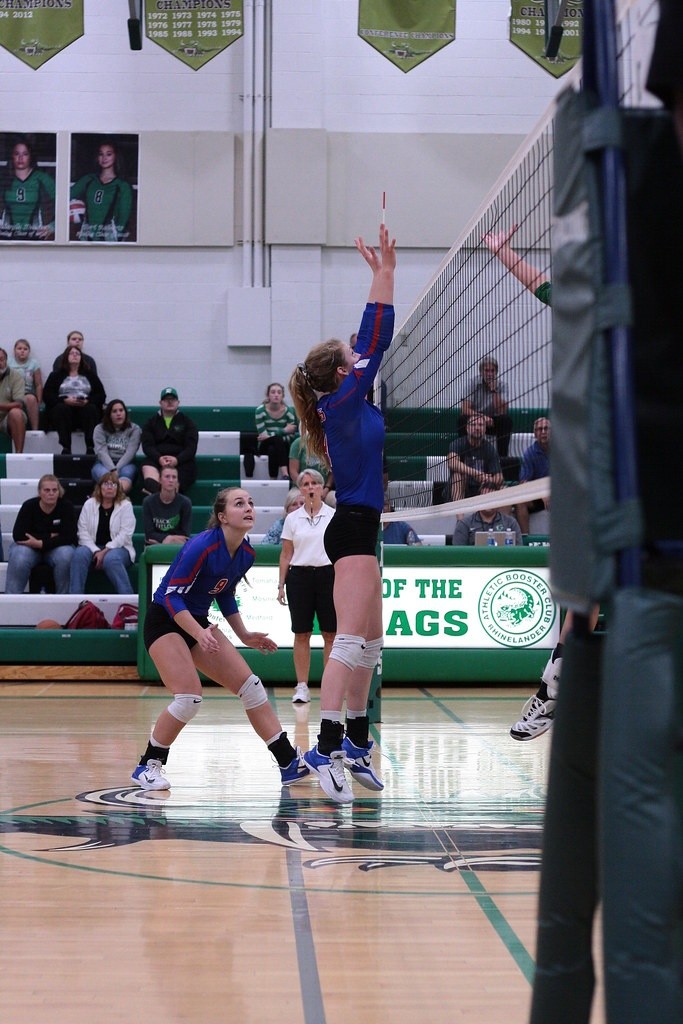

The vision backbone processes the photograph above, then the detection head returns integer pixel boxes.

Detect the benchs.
[0,406,550,630]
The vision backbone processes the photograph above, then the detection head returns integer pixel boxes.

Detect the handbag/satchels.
[113,604,139,631]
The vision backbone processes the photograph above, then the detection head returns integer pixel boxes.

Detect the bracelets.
[492,386,501,392]
[278,584,284,589]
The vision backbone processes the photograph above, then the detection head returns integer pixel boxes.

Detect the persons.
[0,331,198,594]
[131,487,313,791]
[0,140,57,241]
[482,224,600,741]
[288,224,399,803]
[383,356,551,547]
[255,382,336,545]
[277,469,337,703]
[69,139,134,243]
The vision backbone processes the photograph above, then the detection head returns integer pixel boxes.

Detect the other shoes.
[62,446,71,454]
[86,446,95,455]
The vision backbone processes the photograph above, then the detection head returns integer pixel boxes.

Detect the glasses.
[103,481,118,489]
[164,398,177,402]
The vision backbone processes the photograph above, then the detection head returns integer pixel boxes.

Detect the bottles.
[487,528,497,547]
[505,528,514,545]
[406,528,415,547]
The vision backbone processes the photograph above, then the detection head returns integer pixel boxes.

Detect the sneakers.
[341,731,384,791]
[292,683,311,703]
[303,745,354,804]
[278,745,316,786]
[292,702,311,714]
[510,691,555,741]
[131,754,170,790]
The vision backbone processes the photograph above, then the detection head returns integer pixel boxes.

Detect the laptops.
[474,532,515,546]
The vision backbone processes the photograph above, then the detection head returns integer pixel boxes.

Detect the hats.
[161,387,178,401]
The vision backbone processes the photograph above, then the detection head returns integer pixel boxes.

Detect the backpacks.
[63,600,111,629]
